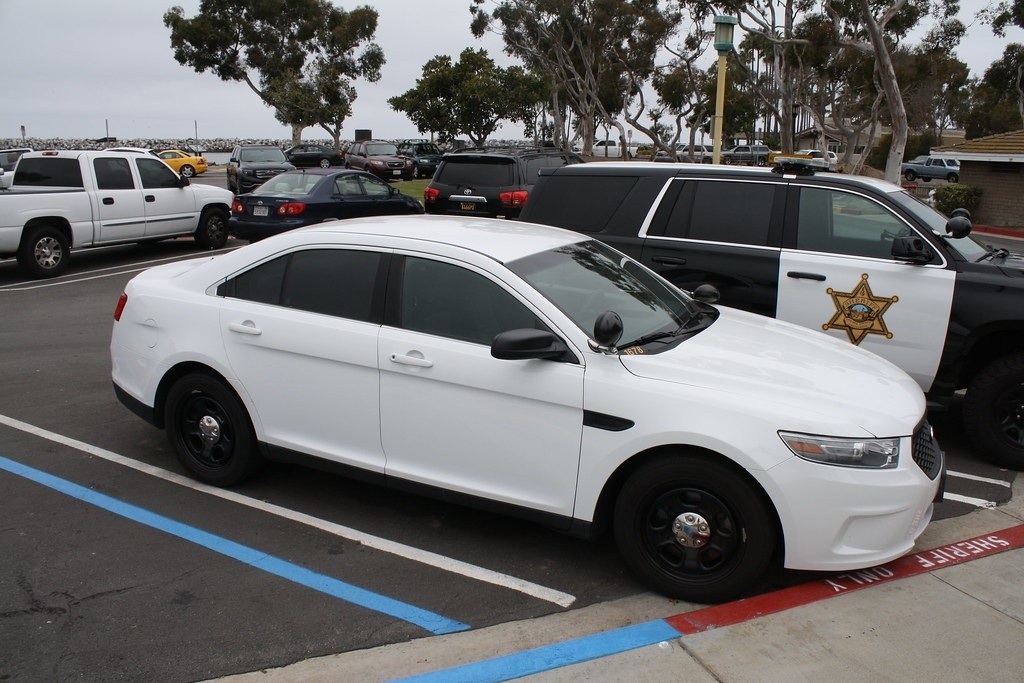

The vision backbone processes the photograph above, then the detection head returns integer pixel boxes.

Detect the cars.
[109,209,943,606]
[0,148,34,190]
[806,151,838,173]
[677,144,714,164]
[158,150,208,177]
[227,166,426,244]
[720,145,774,167]
[283,143,345,168]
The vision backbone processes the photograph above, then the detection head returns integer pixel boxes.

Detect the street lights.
[713,16,737,164]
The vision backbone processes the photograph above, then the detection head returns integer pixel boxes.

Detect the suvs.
[424,146,586,222]
[520,152,1024,473]
[227,144,297,196]
[397,139,443,180]
[103,147,160,159]
[344,139,416,181]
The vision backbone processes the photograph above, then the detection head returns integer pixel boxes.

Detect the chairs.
[111,169,130,188]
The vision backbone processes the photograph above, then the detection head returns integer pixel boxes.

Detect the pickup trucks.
[591,140,639,160]
[0,150,235,280]
[901,154,960,183]
